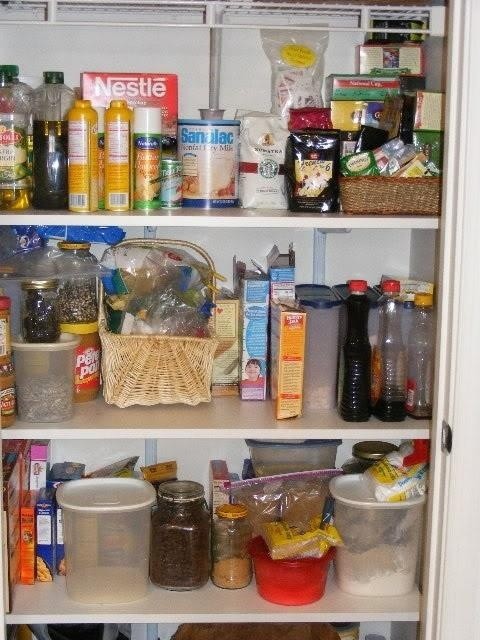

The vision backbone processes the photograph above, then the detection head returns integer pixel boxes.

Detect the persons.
[237,359,268,391]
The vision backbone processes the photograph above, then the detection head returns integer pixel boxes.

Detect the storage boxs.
[0,441,87,615]
[201,236,305,427]
[317,43,443,173]
[79,69,179,141]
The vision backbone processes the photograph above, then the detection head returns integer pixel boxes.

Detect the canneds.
[0,245,101,428]
[342,441,399,474]
[160,160,184,209]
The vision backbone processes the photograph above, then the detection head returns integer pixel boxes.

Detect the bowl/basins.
[250,535,336,605]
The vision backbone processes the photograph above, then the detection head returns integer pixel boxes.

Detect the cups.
[198,107,226,121]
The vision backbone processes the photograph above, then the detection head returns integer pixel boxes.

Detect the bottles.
[1,64,77,213]
[21,278,60,342]
[54,242,99,320]
[104,100,135,209]
[336,280,435,422]
[133,108,162,212]
[340,443,399,479]
[1,295,18,429]
[67,98,101,211]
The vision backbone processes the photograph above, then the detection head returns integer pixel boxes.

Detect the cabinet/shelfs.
[0,0,478,640]
